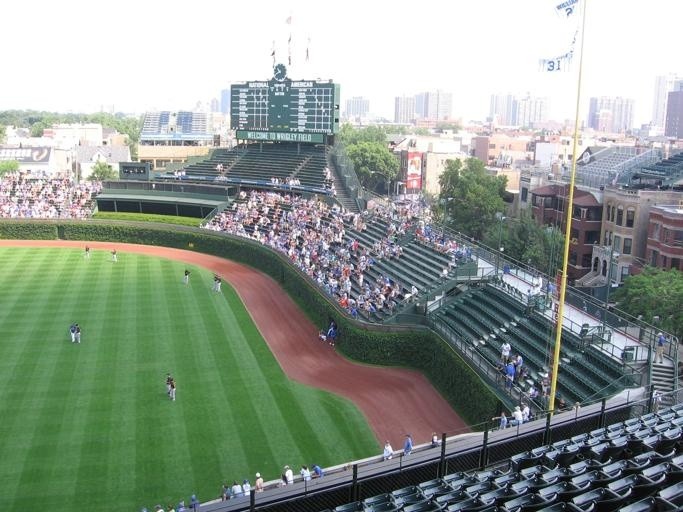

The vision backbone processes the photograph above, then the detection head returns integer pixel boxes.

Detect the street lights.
[370,171,390,197]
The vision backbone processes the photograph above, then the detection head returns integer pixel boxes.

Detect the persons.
[653,332,666,364]
[165,372,176,401]
[275,463,324,488]
[431,432,439,448]
[112,249,117,262]
[491,339,582,430]
[140,494,200,512]
[199,162,473,348]
[404,435,412,455]
[85,245,89,257]
[221,472,264,501]
[174,167,186,177]
[526,274,556,310]
[183,270,190,284]
[0,167,103,220]
[383,440,394,460]
[69,323,81,344]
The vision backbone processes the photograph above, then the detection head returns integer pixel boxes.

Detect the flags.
[538,0,583,77]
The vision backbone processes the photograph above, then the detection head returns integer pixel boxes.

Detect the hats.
[310,463,315,467]
[234,480,238,484]
[192,496,196,501]
[244,480,248,483]
[155,505,162,509]
[224,485,228,488]
[303,465,308,469]
[179,502,184,507]
[284,465,289,468]
[256,472,261,478]
